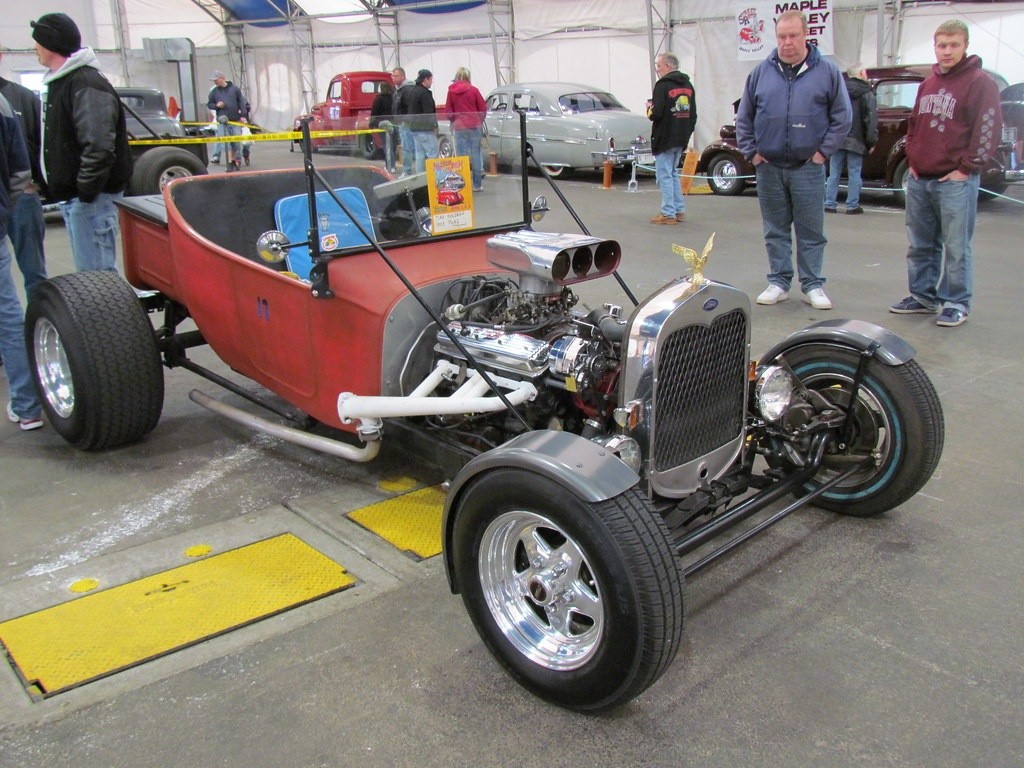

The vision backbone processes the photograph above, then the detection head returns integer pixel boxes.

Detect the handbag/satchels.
[241,126,254,146]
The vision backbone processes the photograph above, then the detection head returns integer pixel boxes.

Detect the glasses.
[30,20,57,32]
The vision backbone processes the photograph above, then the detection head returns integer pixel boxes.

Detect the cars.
[436,81,656,180]
[698,61,1024,209]
[37,86,210,216]
[23,109,947,715]
[292,71,450,161]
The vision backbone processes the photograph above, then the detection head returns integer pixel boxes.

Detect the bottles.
[330,105,340,119]
[646,100,654,118]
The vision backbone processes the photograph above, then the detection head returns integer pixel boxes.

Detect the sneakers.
[651,213,677,225]
[757,283,790,305]
[799,285,832,309]
[675,212,684,220]
[7,401,44,430]
[937,307,967,326]
[890,296,937,314]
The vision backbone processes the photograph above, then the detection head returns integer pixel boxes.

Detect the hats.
[209,72,225,81]
[32,13,81,58]
[415,69,432,86]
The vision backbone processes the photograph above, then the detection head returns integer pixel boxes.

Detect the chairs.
[274,186,378,283]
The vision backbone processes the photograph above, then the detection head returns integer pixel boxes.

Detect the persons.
[735,11,852,309]
[0,77,48,298]
[30,12,135,274]
[0,93,44,430]
[646,53,697,224]
[369,82,399,172]
[410,69,440,173]
[207,71,251,166]
[391,67,414,179]
[825,63,878,215]
[445,67,487,191]
[890,20,1003,327]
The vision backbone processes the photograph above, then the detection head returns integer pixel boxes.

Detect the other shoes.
[211,156,220,163]
[229,159,241,168]
[384,166,395,174]
[846,206,863,214]
[244,154,250,165]
[825,207,837,213]
[397,173,410,180]
[474,186,484,191]
